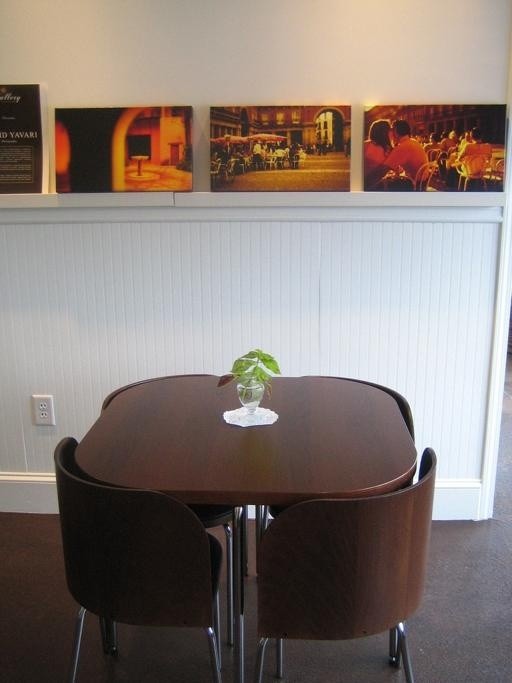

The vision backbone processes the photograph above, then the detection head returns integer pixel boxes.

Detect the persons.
[408,124,492,191]
[209,132,351,189]
[364,117,402,191]
[363,119,429,191]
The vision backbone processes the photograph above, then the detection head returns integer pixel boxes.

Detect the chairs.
[210,154,239,187]
[103,374,253,643]
[370,146,505,191]
[52,437,223,682]
[256,374,416,582]
[252,449,435,681]
[245,143,307,170]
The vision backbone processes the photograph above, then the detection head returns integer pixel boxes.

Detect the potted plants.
[218,350,281,421]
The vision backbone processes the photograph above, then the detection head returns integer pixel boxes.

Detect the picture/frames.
[208,105,354,188]
[362,105,506,189]
[53,107,194,192]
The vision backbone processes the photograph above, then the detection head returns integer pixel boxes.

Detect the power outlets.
[31,394,56,426]
